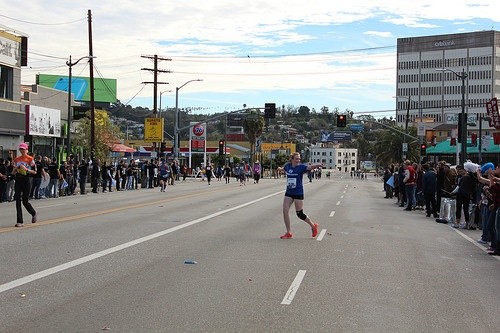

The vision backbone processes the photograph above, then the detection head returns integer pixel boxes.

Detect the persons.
[91,158,188,192]
[350,170,367,180]
[308,169,330,179]
[11,143,37,227]
[277,153,325,239]
[198,160,261,186]
[382,160,500,255]
[1,152,93,203]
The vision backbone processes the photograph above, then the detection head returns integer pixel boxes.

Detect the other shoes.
[311,223,318,237]
[15,223,25,227]
[280,232,293,239]
[32,211,38,223]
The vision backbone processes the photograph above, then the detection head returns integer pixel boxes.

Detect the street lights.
[65,54,98,157]
[478,114,492,167]
[174,78,204,159]
[159,90,174,119]
[392,95,423,123]
[435,67,469,171]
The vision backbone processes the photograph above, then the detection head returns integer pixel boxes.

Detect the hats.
[19,143,28,149]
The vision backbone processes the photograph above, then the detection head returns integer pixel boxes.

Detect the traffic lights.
[336,114,347,128]
[421,144,426,156]
[218,140,224,156]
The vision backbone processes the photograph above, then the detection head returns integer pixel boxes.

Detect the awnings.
[426,136,500,153]
